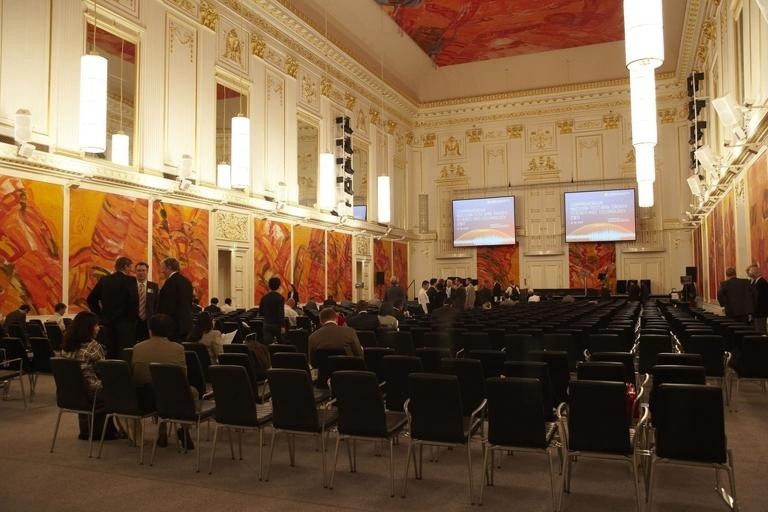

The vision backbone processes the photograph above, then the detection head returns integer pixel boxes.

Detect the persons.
[47,302,66,338]
[561,291,575,304]
[204,297,220,312]
[323,296,335,308]
[305,296,318,313]
[57,311,120,441]
[346,299,378,332]
[286,285,298,302]
[258,277,284,341]
[191,296,204,314]
[377,300,398,332]
[282,297,298,327]
[716,267,755,325]
[417,274,521,317]
[601,284,610,297]
[745,264,768,334]
[639,280,650,303]
[155,257,192,344]
[190,313,225,365]
[219,298,236,314]
[628,282,641,298]
[306,308,363,368]
[129,313,196,451]
[86,257,139,361]
[384,274,406,313]
[526,288,540,302]
[133,261,158,341]
[4,304,32,352]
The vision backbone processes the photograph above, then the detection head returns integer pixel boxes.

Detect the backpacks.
[510,286,519,301]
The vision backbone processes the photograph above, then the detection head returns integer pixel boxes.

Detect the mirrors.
[216,74,255,191]
[83,12,142,172]
[351,134,370,225]
[296,111,323,213]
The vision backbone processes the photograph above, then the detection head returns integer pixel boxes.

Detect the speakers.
[617,280,626,295]
[695,144,715,171]
[687,174,702,196]
[641,280,650,295]
[377,272,384,285]
[686,267,696,282]
[712,93,743,126]
[628,280,638,292]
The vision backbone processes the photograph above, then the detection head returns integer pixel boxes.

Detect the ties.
[139,283,146,321]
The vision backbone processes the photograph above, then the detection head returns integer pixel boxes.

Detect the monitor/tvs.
[353,205,367,221]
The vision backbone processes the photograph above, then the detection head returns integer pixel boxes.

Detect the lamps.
[418,52,429,234]
[80,2,107,154]
[111,40,130,165]
[319,0,337,212]
[231,1,252,191]
[216,87,231,191]
[624,0,752,208]
[176,156,193,190]
[377,0,390,223]
[14,113,35,159]
